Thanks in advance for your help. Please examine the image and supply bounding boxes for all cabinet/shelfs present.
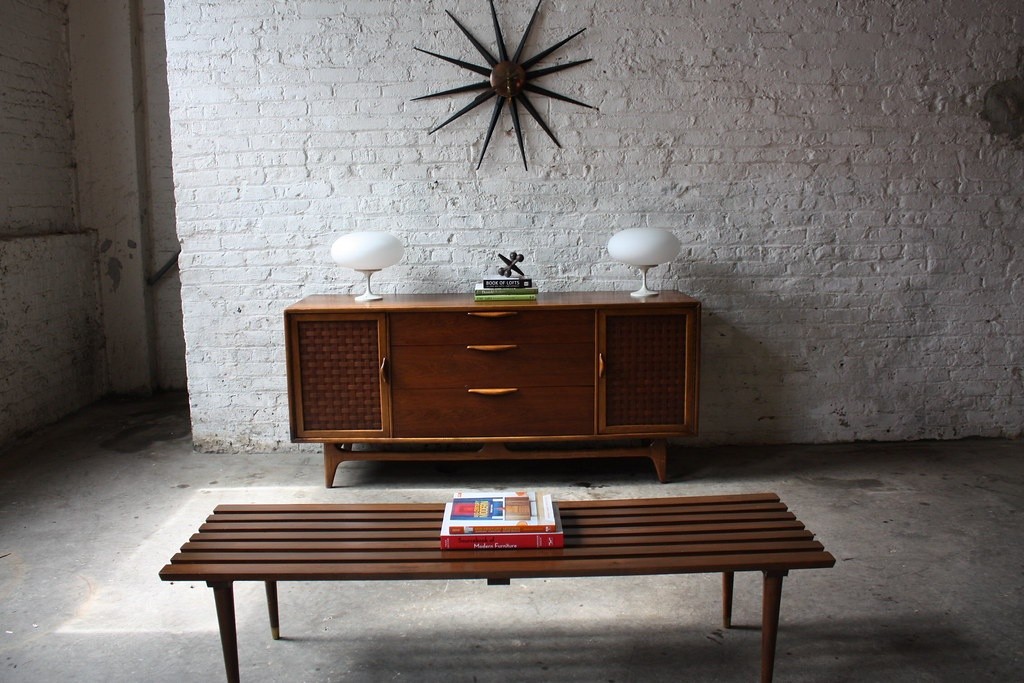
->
[283,291,703,488]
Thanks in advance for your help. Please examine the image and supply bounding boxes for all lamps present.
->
[608,228,681,297]
[331,231,404,302]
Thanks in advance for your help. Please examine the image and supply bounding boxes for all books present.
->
[474,275,539,302]
[440,491,565,550]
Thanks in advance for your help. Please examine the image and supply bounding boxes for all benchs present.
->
[159,492,835,683]
